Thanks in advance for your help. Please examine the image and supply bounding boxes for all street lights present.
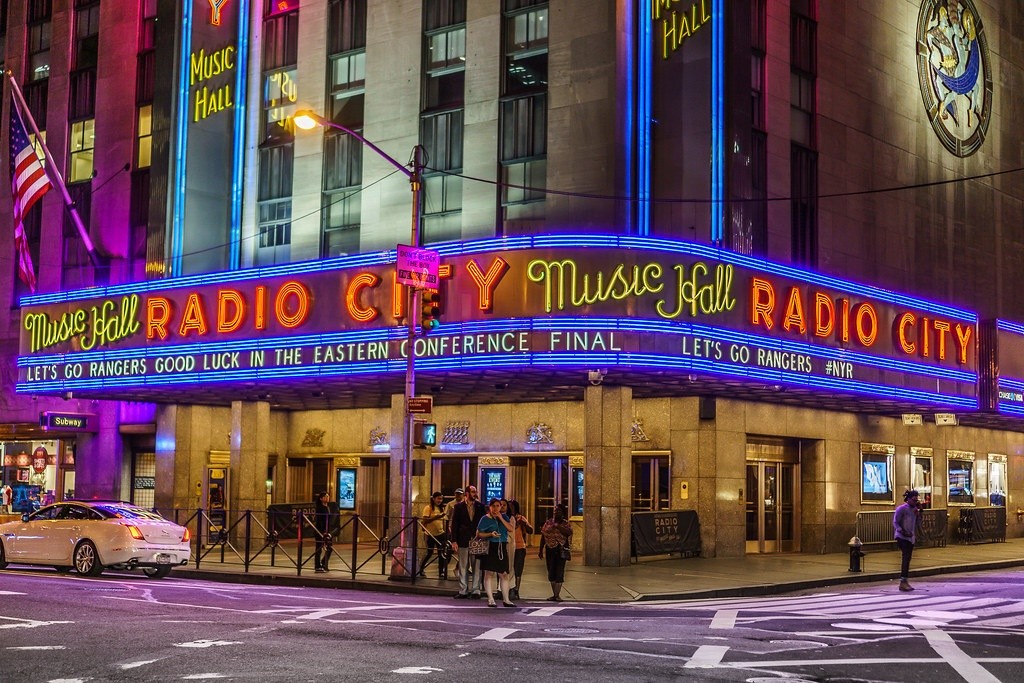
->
[292,107,425,583]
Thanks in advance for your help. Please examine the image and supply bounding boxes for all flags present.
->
[8,90,55,293]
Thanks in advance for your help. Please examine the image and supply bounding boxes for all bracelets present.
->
[919,509,924,512]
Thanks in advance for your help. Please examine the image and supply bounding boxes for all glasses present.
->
[909,497,919,503]
[470,490,479,493]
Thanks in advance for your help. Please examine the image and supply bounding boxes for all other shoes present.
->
[419,568,426,576]
[322,563,329,571]
[315,567,327,573]
[453,593,468,599]
[554,595,563,602]
[438,566,445,577]
[453,568,460,581]
[469,566,472,573]
[898,580,914,591]
[502,601,518,607]
[487,600,497,607]
[546,595,555,601]
[471,593,482,599]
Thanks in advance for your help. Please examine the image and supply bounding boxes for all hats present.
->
[454,488,464,494]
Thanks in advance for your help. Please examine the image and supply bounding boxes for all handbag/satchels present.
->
[561,548,572,562]
[468,532,490,556]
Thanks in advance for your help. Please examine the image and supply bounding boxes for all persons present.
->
[476,498,517,607]
[315,491,335,572]
[451,486,482,599]
[497,499,516,598]
[892,491,923,591]
[444,488,464,578]
[419,492,444,577]
[537,503,574,601]
[508,499,534,599]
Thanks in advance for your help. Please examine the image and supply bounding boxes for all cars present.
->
[0,501,192,579]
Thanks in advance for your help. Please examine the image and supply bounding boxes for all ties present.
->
[466,500,474,521]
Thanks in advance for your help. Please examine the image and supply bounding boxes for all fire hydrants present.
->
[847,535,867,573]
